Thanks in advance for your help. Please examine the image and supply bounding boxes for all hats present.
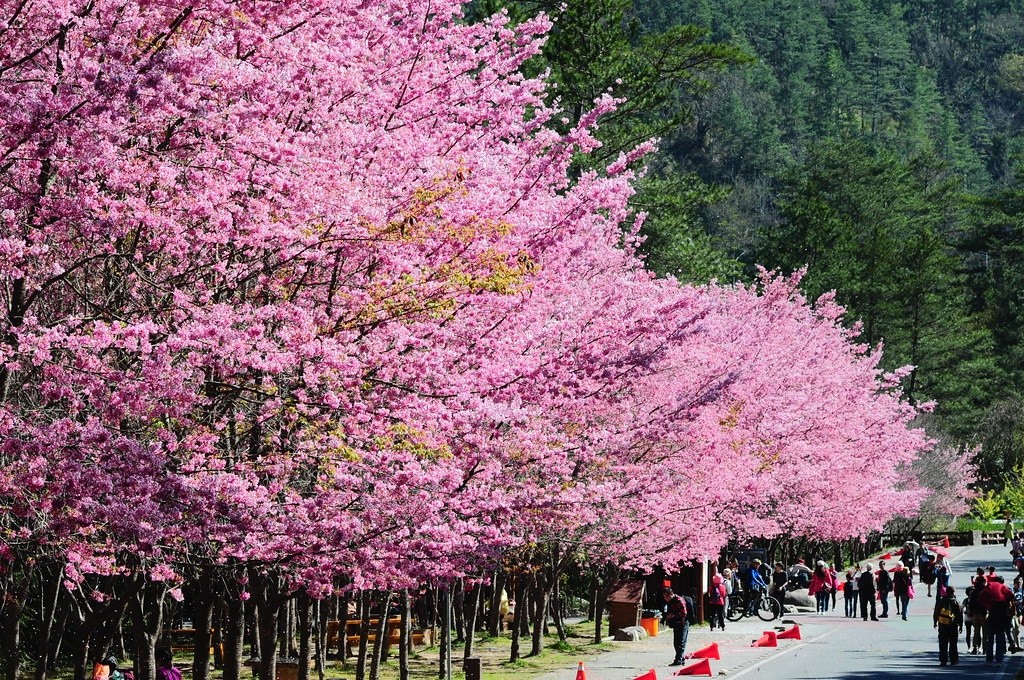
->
[866,563,873,570]
[753,558,759,562]
[986,565,995,572]
[902,567,911,575]
[799,559,805,563]
[722,568,731,575]
[817,560,824,566]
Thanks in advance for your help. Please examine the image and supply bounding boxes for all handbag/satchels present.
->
[908,586,914,599]
[824,583,830,593]
[91,662,110,680]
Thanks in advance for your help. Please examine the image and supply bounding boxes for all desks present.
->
[323,617,417,661]
[161,629,226,666]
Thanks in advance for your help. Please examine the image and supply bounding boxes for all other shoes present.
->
[864,617,867,621]
[878,614,888,618]
[871,616,879,621]
[902,616,907,621]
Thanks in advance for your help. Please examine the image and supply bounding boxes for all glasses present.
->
[753,562,760,565]
[878,564,883,566]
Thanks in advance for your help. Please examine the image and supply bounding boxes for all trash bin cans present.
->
[639,609,659,636]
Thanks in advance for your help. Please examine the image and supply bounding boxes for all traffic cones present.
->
[894,548,904,555]
[874,570,880,574]
[633,668,658,680]
[887,566,896,573]
[943,536,950,548]
[879,552,891,560]
[576,662,588,680]
[776,624,802,640]
[750,630,778,647]
[684,642,721,660]
[672,656,712,677]
[836,581,845,591]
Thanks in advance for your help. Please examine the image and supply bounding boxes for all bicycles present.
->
[725,586,781,622]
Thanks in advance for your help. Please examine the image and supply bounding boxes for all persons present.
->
[809,560,839,621]
[661,587,690,666]
[898,541,953,599]
[492,583,509,633]
[708,573,727,631]
[786,559,815,589]
[93,650,182,680]
[1004,518,1024,569]
[933,556,1024,666]
[718,558,788,629]
[843,560,915,621]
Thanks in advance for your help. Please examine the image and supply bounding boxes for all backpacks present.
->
[786,564,797,577]
[888,577,894,591]
[709,583,721,602]
[937,600,955,625]
[918,550,929,564]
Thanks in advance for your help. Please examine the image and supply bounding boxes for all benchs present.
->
[330,633,424,656]
[171,647,226,665]
